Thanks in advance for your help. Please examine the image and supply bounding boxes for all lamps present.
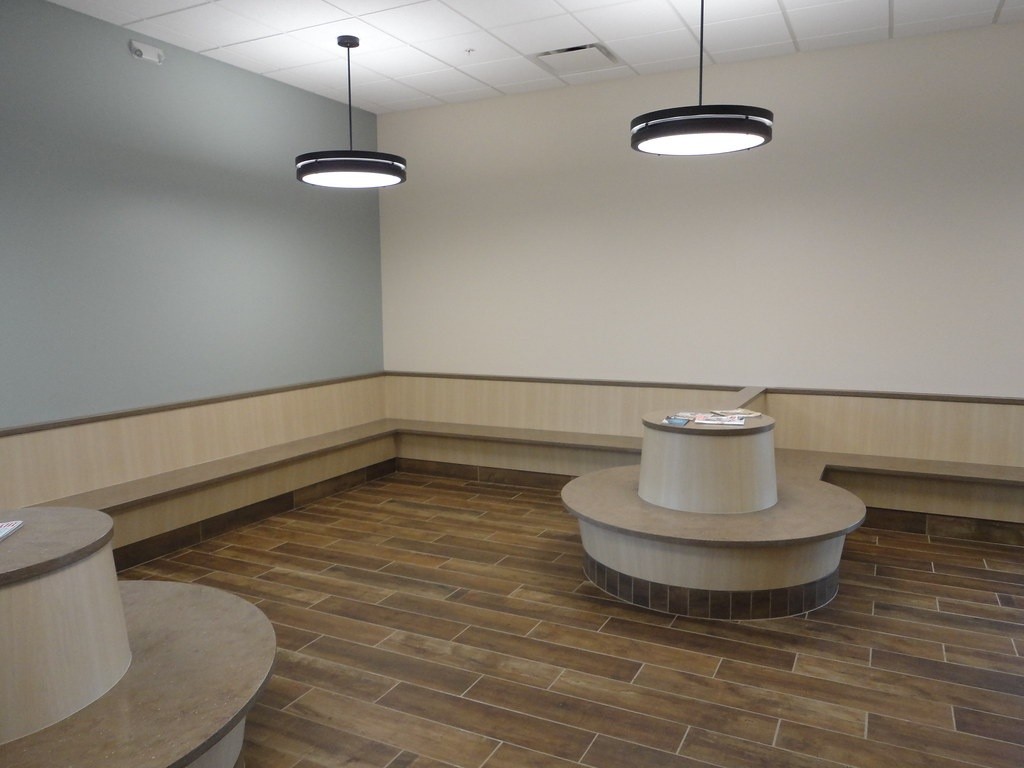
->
[294,35,406,188]
[629,0,773,154]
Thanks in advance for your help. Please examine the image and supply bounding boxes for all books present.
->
[0,521,24,544]
[656,407,763,426]
